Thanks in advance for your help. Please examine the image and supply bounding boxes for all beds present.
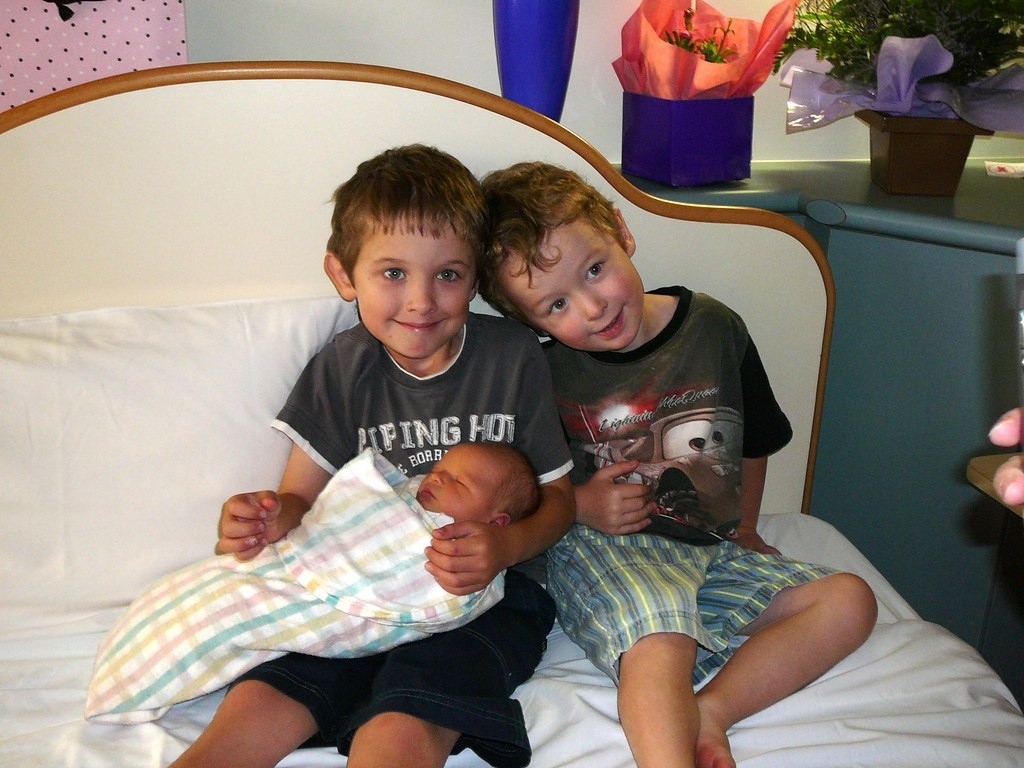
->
[0,62,1024,768]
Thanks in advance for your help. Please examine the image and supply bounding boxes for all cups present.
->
[1017,236,1024,407]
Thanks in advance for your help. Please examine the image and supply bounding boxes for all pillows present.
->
[0,296,362,606]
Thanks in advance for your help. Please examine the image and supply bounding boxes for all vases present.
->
[854,107,995,199]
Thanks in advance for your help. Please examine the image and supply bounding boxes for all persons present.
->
[480,162,879,767]
[989,407,1024,506]
[83,441,541,727]
[169,142,578,767]
